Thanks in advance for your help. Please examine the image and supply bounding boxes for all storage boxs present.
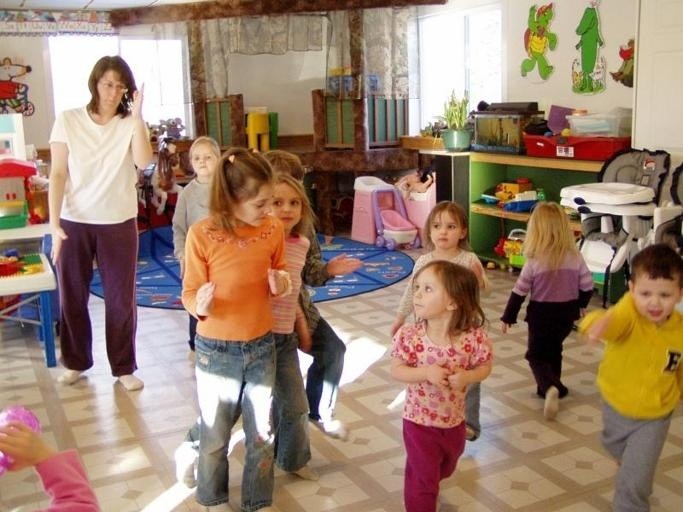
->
[521,129,632,162]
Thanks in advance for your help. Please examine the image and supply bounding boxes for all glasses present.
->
[94,78,130,94]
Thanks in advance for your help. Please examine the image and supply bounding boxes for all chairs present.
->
[639,164,682,272]
[559,147,670,306]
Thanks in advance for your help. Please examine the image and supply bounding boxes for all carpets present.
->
[90,226,415,311]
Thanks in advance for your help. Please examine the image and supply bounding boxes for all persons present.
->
[576,241,683,511]
[497,202,595,424]
[47,56,155,390]
[171,136,363,512]
[0,420,101,512]
[390,200,493,442]
[390,259,493,511]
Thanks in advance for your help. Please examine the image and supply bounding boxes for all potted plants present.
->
[443,89,472,151]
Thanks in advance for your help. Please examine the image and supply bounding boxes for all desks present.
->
[0,223,56,270]
[0,251,57,367]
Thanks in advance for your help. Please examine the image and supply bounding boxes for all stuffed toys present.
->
[146,118,185,216]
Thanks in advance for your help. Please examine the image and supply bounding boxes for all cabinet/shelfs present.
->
[468,153,630,305]
